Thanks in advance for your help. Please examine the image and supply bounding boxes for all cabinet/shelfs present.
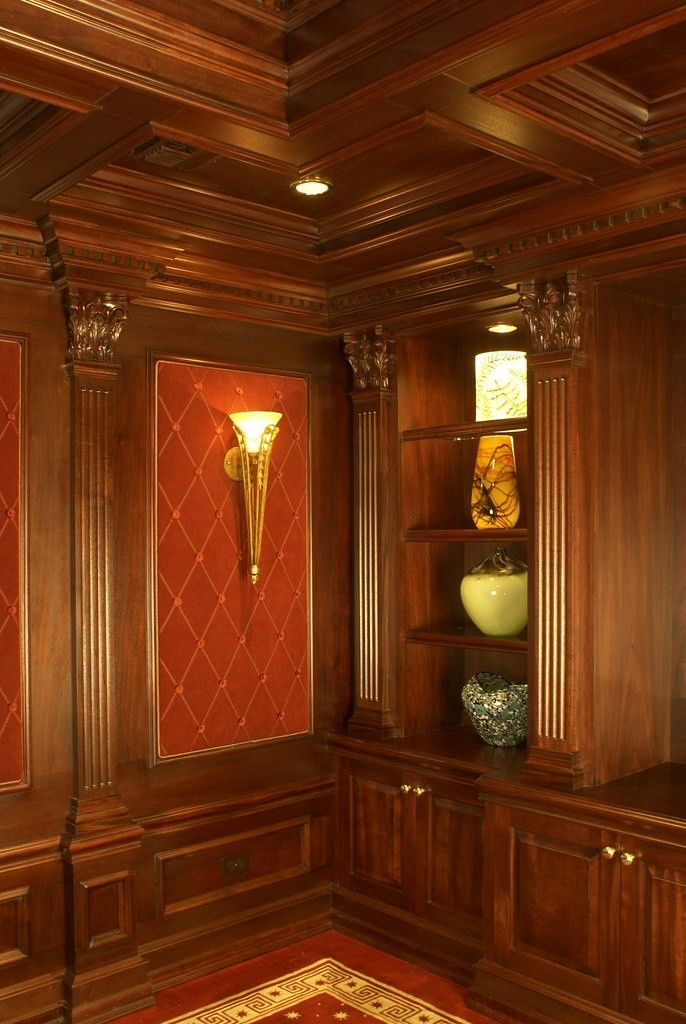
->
[401,418,530,653]
[324,729,526,986]
[466,762,686,1023]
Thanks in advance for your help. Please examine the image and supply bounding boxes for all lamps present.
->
[223,410,284,586]
